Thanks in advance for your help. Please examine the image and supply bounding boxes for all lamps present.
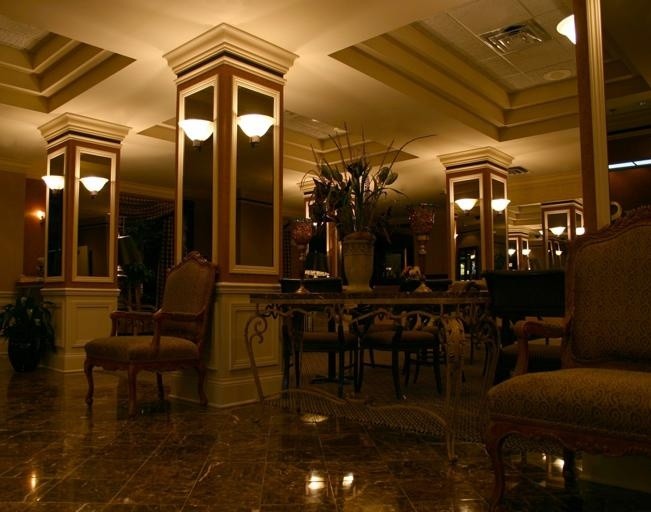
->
[455,198,478,215]
[508,226,585,256]
[80,176,110,199]
[178,118,214,149]
[556,14,576,45]
[40,175,65,196]
[236,113,274,142]
[608,156,651,173]
[491,198,511,215]
[37,210,46,226]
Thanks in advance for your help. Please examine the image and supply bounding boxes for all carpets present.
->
[263,365,651,458]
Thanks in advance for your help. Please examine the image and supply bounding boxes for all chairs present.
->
[279,268,569,399]
[84,252,220,421]
[482,205,651,512]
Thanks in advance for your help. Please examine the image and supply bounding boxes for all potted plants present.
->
[299,126,439,285]
[1,287,54,370]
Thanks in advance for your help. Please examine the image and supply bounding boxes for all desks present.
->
[244,292,487,422]
